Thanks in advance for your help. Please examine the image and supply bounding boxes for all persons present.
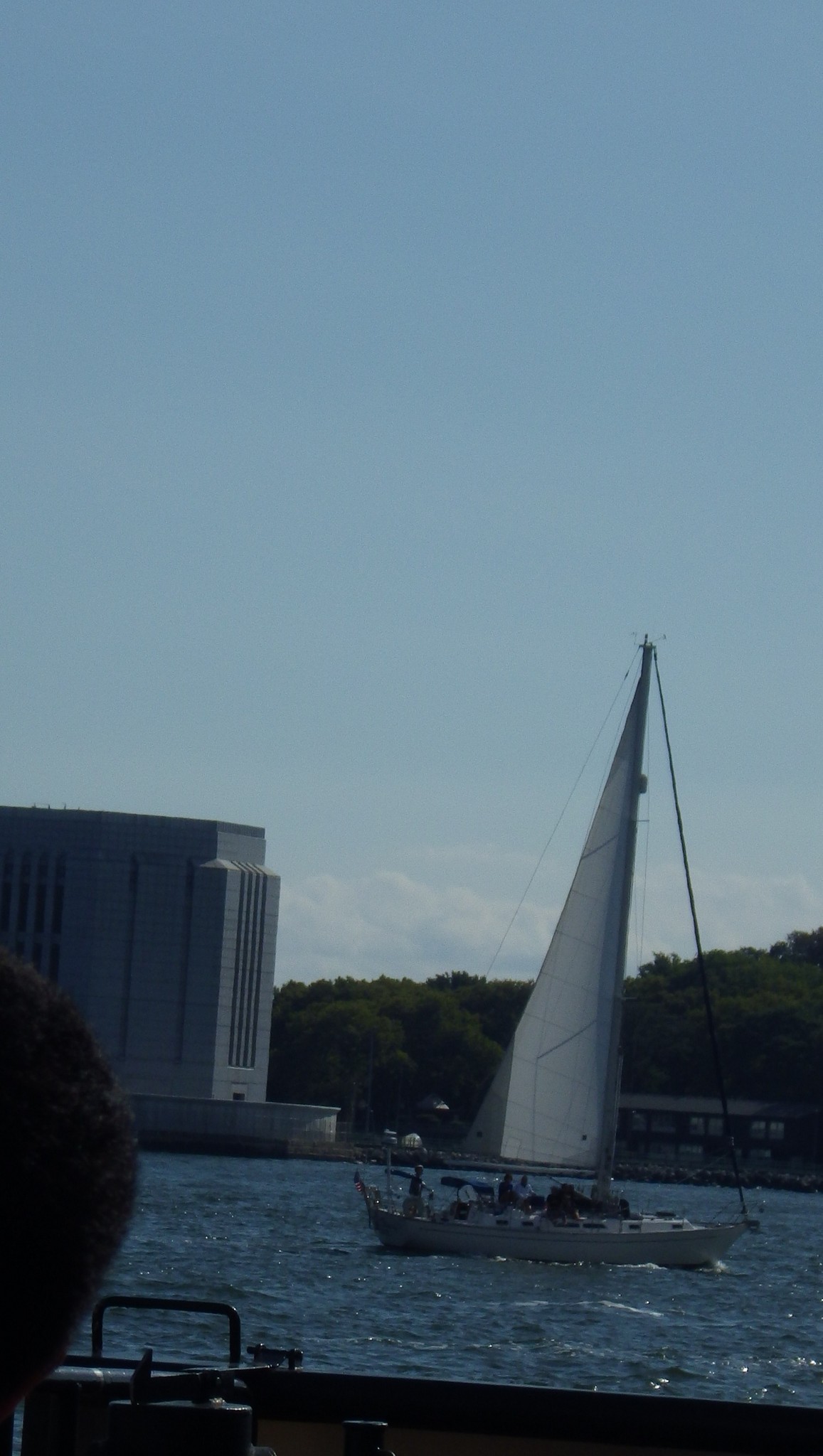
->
[396,1159,630,1229]
[0,943,142,1426]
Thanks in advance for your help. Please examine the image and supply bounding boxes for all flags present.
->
[353,1171,362,1192]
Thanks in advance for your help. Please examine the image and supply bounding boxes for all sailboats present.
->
[354,631,762,1270]
